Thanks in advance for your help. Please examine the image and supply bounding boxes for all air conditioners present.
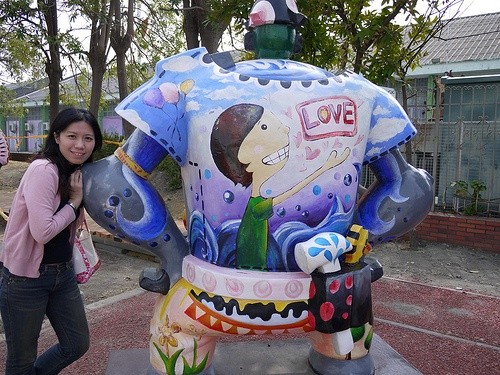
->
[9,123,17,131]
[25,122,33,131]
[42,122,50,130]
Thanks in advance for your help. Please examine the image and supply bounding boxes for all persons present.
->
[0,108,105,375]
